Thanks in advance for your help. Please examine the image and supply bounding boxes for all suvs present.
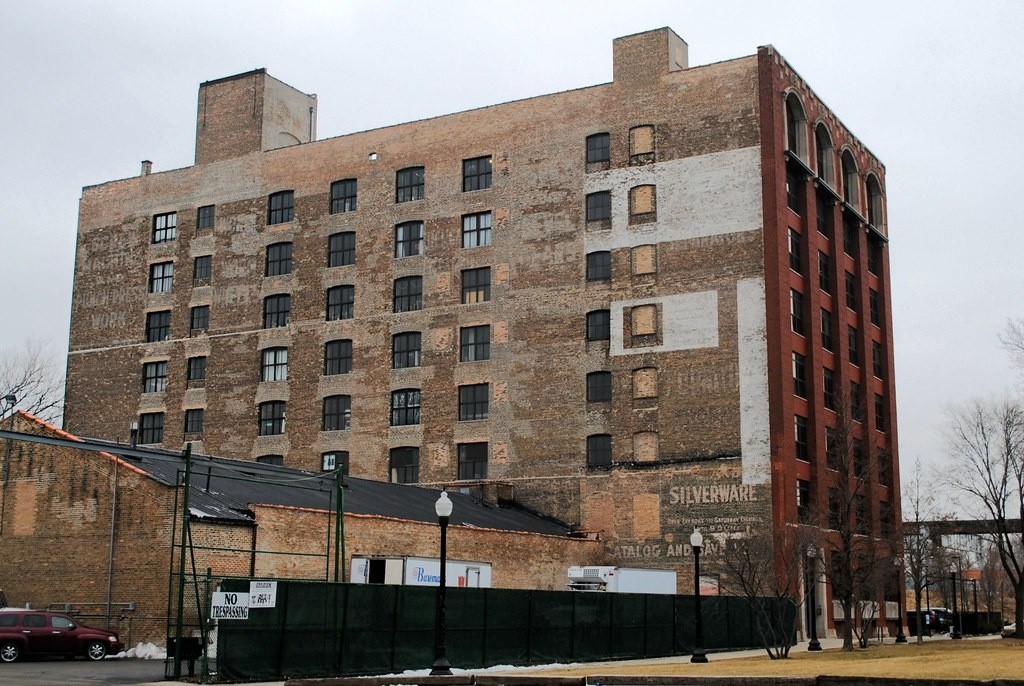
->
[0,607,126,664]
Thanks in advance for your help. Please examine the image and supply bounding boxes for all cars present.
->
[897,606,953,643]
[1001,620,1024,638]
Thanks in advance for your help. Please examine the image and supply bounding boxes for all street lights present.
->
[428,490,453,675]
[805,543,823,651]
[690,528,709,663]
[949,561,963,639]
